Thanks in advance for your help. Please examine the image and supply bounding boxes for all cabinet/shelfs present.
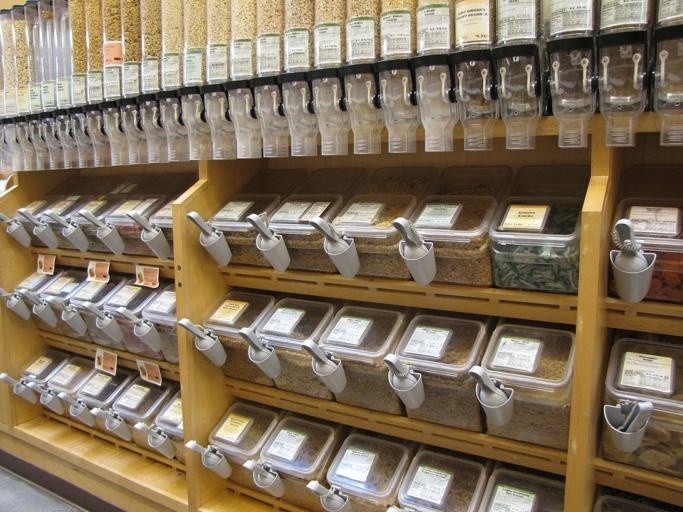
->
[1,106,683,512]
